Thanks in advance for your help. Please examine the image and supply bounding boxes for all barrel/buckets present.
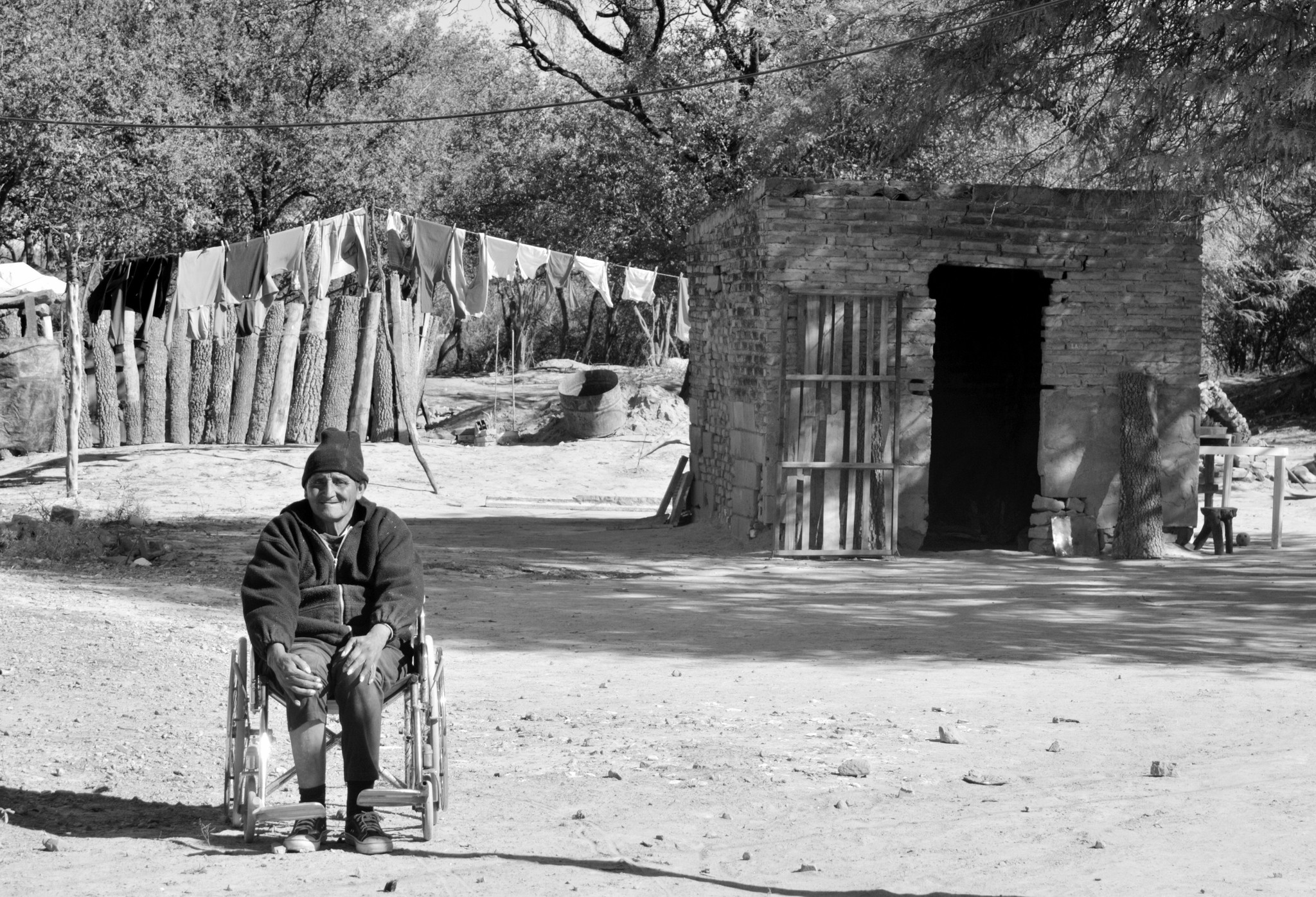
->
[558,369,626,438]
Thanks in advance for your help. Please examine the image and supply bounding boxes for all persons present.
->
[242,427,424,854]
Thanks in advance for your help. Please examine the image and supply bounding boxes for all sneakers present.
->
[283,815,328,852]
[345,811,393,855]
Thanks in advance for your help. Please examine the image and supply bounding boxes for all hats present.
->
[301,427,369,489]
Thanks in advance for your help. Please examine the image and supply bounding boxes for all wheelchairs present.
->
[224,595,448,840]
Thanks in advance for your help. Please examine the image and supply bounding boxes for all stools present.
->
[1193,507,1238,555]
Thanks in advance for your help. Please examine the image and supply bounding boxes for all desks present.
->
[1197,447,1289,548]
